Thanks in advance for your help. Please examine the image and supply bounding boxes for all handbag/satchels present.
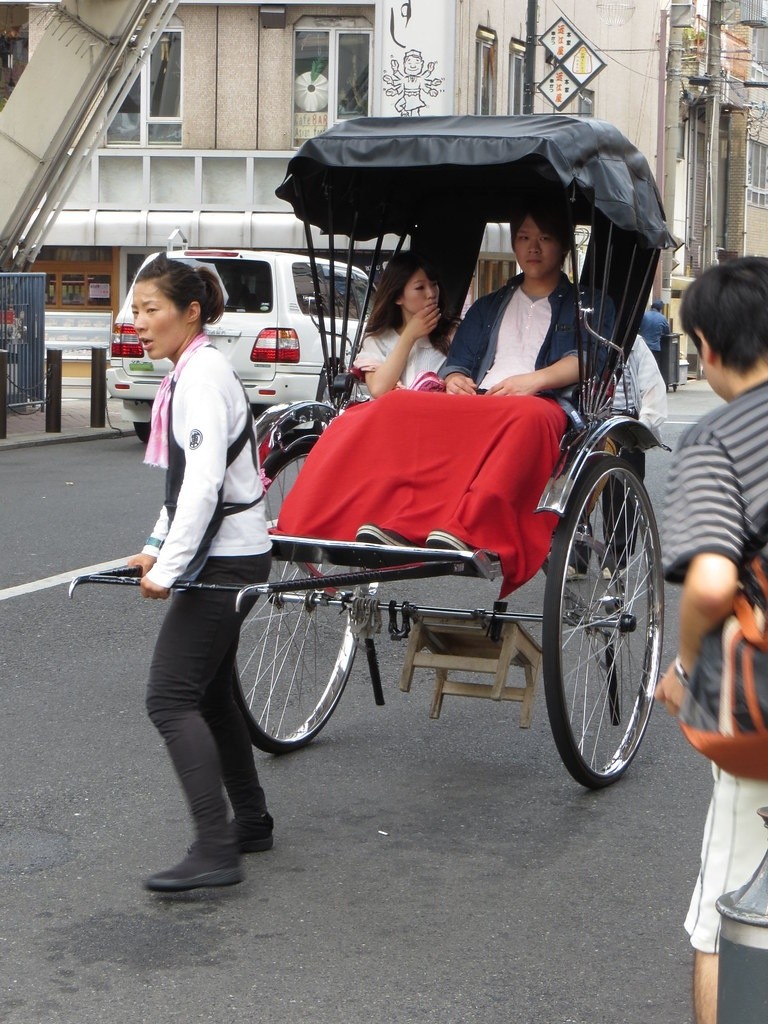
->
[677,519,767,780]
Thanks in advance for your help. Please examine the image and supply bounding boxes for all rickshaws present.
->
[69,111,672,795]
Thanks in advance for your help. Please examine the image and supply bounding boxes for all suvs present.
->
[103,249,381,445]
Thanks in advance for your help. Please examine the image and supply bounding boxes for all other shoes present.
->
[187,814,274,852]
[355,523,418,548]
[565,565,587,581]
[603,567,626,579]
[426,530,472,552]
[143,843,244,891]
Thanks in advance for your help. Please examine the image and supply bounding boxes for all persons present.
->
[354,251,457,399]
[355,199,582,556]
[543,332,667,581]
[650,256,768,1024]
[640,300,670,367]
[129,254,274,894]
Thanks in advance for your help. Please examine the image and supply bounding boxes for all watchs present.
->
[675,657,688,686]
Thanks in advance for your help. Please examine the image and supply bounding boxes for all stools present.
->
[398,615,544,730]
